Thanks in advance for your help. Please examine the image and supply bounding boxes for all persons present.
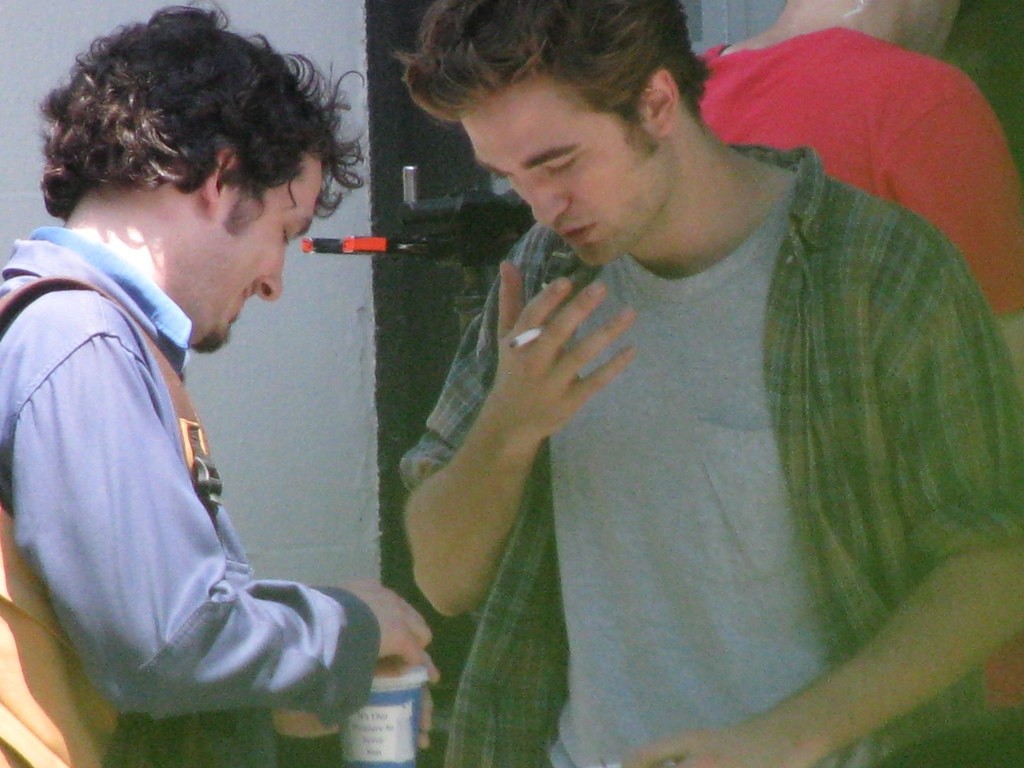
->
[698,0,1024,768]
[401,1,1022,768]
[0,0,439,768]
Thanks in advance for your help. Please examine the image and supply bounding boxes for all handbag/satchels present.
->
[0,278,222,768]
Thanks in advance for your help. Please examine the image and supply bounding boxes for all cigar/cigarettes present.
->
[511,323,549,349]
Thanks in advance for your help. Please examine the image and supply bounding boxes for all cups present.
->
[339,666,426,768]
